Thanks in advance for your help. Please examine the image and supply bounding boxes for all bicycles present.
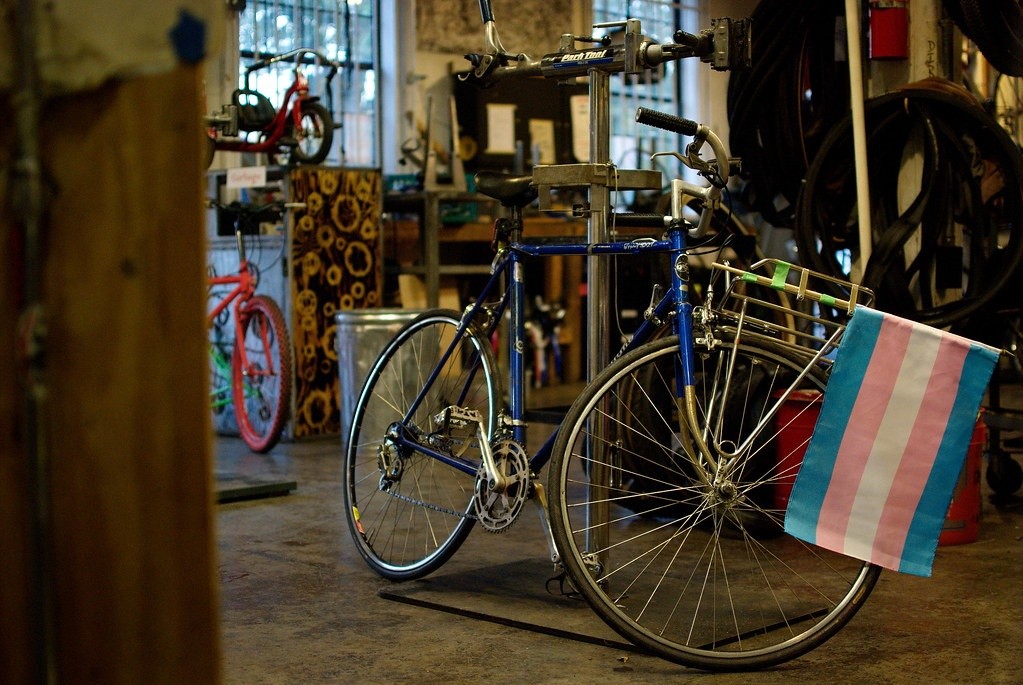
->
[203,195,303,452]
[346,108,888,668]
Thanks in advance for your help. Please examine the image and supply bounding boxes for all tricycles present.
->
[204,48,337,170]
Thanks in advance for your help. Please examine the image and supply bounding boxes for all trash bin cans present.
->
[936,407,988,546]
[772,388,825,516]
[332,307,443,460]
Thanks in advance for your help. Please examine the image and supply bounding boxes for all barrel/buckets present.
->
[336,303,449,458]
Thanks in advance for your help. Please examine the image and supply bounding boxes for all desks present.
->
[379,212,667,385]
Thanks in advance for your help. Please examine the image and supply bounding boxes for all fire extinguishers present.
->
[868,0,910,59]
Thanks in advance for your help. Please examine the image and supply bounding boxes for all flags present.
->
[783,306,999,579]
